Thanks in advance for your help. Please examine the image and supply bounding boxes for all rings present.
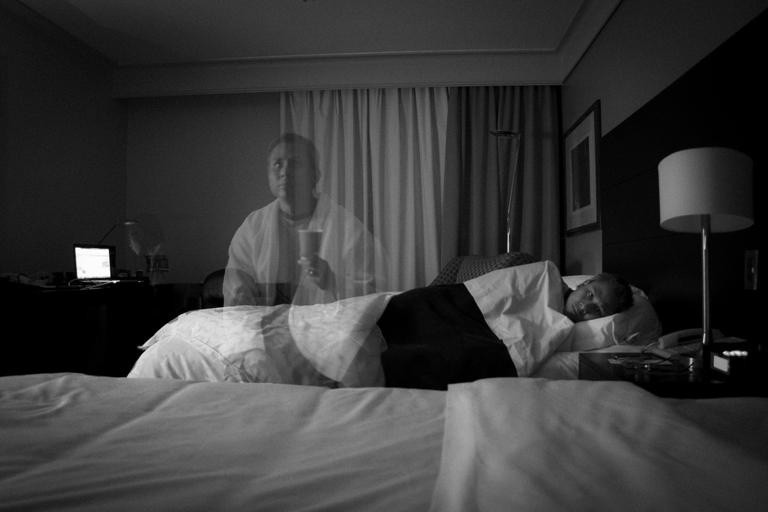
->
[309,268,317,276]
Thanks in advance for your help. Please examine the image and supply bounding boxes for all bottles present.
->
[144,224,170,285]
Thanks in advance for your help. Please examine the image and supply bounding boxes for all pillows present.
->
[561,275,661,352]
[562,99,602,237]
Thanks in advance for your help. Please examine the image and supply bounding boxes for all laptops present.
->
[73,244,145,285]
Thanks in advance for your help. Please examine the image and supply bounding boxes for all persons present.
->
[221,131,390,386]
[560,272,634,322]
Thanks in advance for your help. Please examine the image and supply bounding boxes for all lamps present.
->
[657,148,753,369]
[98,220,138,245]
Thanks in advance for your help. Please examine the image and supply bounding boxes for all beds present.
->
[0,372,768,512]
[126,260,663,389]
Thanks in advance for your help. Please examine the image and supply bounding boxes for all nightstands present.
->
[578,352,768,398]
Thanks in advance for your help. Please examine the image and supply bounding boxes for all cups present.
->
[295,228,323,260]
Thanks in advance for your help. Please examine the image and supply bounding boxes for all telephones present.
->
[647,328,746,359]
